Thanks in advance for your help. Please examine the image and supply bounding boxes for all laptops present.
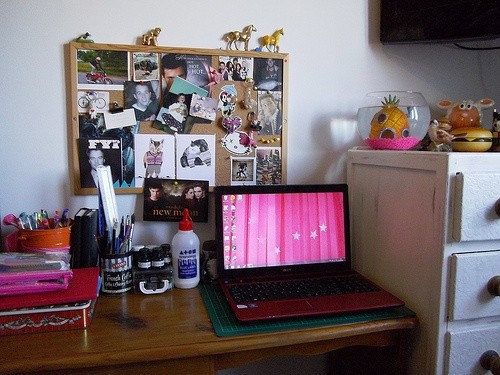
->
[214,184,407,322]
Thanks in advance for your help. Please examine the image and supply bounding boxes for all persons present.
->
[145,181,207,217]
[84,146,117,189]
[259,93,281,136]
[87,55,105,76]
[260,59,280,90]
[161,92,189,134]
[130,82,156,120]
[220,57,248,82]
[159,52,187,89]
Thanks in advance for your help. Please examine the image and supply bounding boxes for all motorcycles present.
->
[86,70,114,85]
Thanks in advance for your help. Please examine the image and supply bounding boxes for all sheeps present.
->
[435,98,495,128]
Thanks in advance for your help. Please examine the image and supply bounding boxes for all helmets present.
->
[96,57,101,60]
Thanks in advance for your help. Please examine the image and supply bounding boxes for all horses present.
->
[226,24,257,51]
[259,28,285,53]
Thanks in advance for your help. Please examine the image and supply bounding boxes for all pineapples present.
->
[367,95,409,140]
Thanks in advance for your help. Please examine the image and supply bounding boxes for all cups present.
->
[18,218,73,254]
[100,247,133,297]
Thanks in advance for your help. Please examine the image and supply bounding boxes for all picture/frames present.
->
[67,41,286,197]
[143,177,209,223]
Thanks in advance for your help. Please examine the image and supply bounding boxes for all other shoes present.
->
[91,73,96,75]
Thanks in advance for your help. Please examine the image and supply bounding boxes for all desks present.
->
[0,287,421,375]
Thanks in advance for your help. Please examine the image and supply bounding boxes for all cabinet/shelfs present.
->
[346,150,500,375]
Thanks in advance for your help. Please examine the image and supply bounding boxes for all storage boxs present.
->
[0,292,99,337]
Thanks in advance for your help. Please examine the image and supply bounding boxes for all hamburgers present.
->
[449,128,493,151]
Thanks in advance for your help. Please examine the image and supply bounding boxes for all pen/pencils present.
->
[2,207,73,229]
[92,213,136,254]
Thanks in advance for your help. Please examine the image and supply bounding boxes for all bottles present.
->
[201,240,218,284]
[132,244,171,270]
[171,208,200,288]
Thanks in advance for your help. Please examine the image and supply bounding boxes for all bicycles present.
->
[78,92,106,109]
